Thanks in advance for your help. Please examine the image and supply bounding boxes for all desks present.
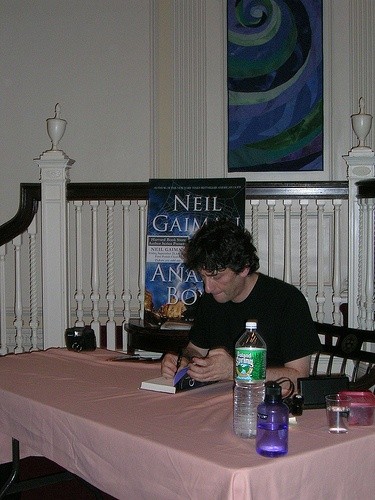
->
[0,339,375,500]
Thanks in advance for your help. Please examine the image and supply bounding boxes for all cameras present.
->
[65,327,96,353]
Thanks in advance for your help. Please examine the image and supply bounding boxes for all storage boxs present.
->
[338,391,375,427]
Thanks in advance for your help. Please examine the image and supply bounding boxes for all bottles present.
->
[233,322,267,437]
[256,379,294,457]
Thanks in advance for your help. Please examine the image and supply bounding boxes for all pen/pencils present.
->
[174,353,182,376]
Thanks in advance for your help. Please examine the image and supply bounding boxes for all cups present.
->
[325,394,352,434]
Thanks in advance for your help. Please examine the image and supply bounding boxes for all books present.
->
[141,357,219,395]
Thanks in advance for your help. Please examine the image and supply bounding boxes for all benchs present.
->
[309,321,375,396]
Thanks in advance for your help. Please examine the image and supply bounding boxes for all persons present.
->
[161,218,323,399]
